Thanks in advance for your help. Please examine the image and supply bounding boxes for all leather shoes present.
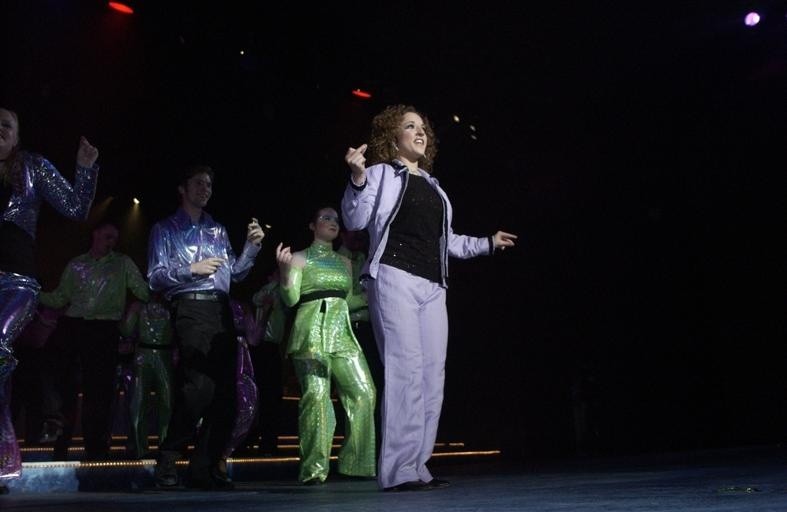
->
[419,477,451,490]
[382,481,433,493]
[153,465,178,487]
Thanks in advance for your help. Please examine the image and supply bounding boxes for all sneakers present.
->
[188,460,235,493]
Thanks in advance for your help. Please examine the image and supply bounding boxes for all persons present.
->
[273,206,380,484]
[337,104,519,493]
[15,215,376,478]
[146,157,269,490]
[0,102,102,496]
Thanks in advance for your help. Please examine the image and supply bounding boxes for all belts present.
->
[173,290,229,303]
